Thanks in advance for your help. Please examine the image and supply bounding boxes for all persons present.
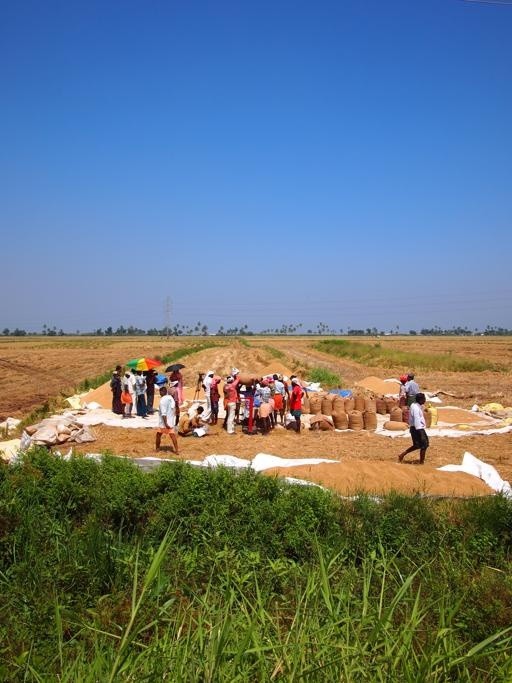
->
[109,365,309,456]
[398,376,409,408]
[397,392,430,464]
[403,372,421,408]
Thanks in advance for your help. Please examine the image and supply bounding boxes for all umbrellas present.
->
[124,357,164,375]
